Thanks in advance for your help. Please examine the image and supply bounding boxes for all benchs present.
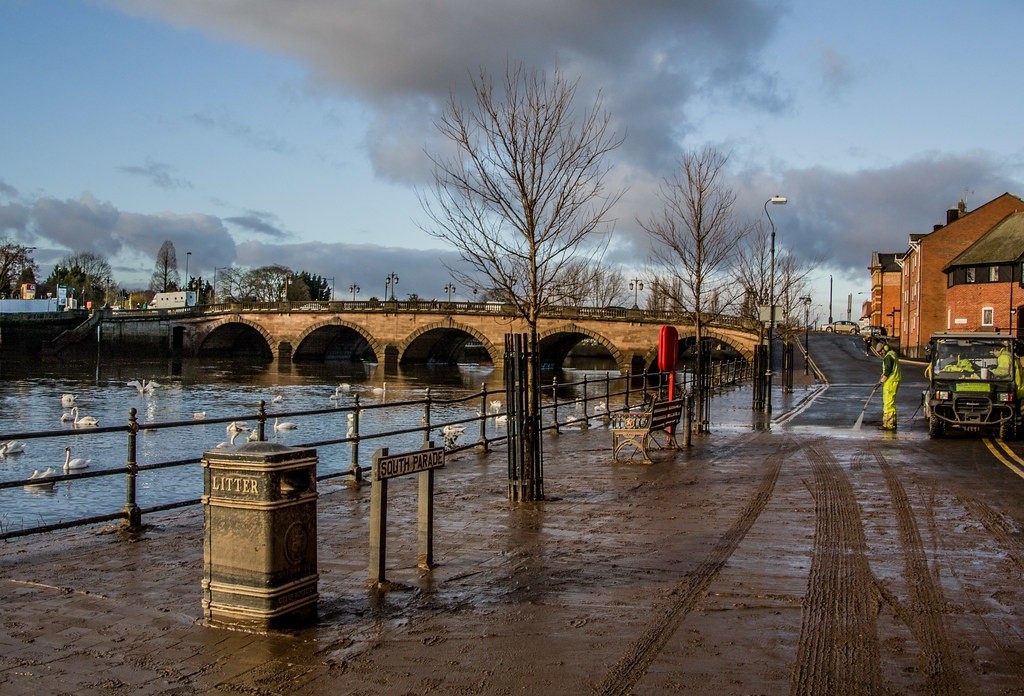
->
[613,389,689,464]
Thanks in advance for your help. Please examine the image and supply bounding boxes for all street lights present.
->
[629,277,644,310]
[803,294,812,375]
[349,283,360,301]
[443,282,456,303]
[20,246,36,283]
[186,252,192,290]
[213,267,233,303]
[322,276,334,300]
[763,195,788,389]
[386,272,400,301]
[280,272,294,301]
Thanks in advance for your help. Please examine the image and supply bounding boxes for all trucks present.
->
[922,331,1019,442]
[146,291,196,311]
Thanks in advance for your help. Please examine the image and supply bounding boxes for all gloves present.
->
[874,381,883,390]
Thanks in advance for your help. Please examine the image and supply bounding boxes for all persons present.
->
[934,352,975,376]
[136,302,148,314]
[876,341,901,431]
[988,344,1024,443]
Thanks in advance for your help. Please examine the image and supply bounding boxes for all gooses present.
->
[193,411,207,420]
[216,421,268,449]
[70,406,99,425]
[329,382,351,399]
[345,402,364,444]
[565,396,643,426]
[61,406,79,422]
[443,399,507,448]
[0,440,26,454]
[62,393,79,408]
[63,447,91,469]
[25,467,57,487]
[127,379,161,397]
[273,418,298,430]
[273,395,283,403]
[373,381,387,391]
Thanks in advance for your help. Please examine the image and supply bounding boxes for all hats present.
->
[874,342,885,352]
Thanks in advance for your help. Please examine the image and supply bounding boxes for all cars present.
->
[821,321,860,336]
[859,325,887,337]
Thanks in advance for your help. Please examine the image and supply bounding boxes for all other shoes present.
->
[878,425,895,430]
